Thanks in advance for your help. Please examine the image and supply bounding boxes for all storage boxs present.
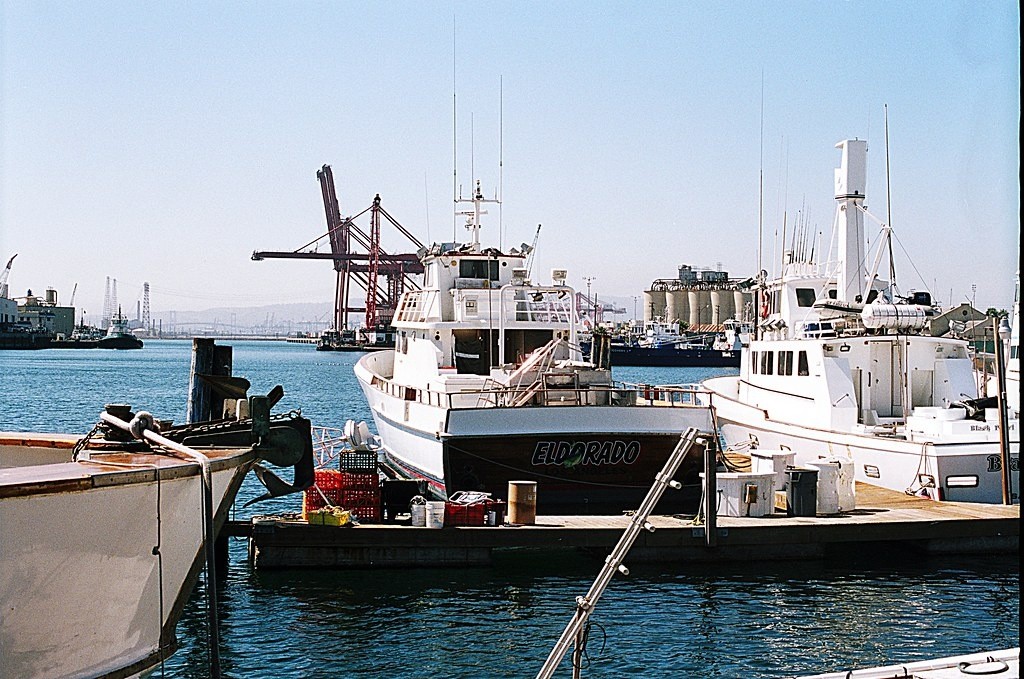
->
[302,450,382,524]
[384,480,428,521]
[444,501,505,527]
[699,472,776,515]
[751,445,795,491]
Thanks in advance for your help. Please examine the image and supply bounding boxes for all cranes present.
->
[251,164,429,330]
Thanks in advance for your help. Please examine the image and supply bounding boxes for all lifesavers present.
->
[760,291,769,317]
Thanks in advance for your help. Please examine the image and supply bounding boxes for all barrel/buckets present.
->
[425,500,445,528]
[507,480,537,526]
[487,501,506,526]
[784,455,856,518]
[412,505,426,526]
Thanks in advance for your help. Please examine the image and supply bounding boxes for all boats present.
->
[0,386,315,679]
[354,12,1019,517]
[51,304,144,349]
